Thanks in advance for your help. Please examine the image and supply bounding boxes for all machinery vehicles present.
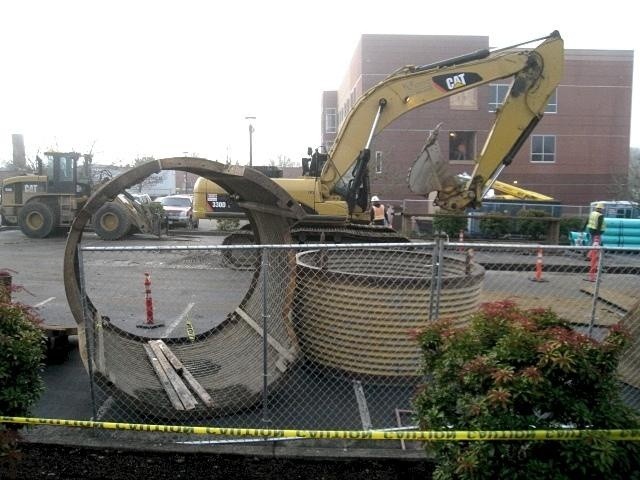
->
[452,172,557,202]
[0,147,169,240]
[194,29,566,272]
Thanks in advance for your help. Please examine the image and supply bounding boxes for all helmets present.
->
[371,196,381,202]
[595,202,604,209]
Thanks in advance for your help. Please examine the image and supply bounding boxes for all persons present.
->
[579,203,609,262]
[457,139,467,160]
[367,194,390,228]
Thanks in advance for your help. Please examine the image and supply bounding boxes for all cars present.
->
[159,192,199,231]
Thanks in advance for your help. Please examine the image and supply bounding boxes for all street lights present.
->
[243,116,257,168]
